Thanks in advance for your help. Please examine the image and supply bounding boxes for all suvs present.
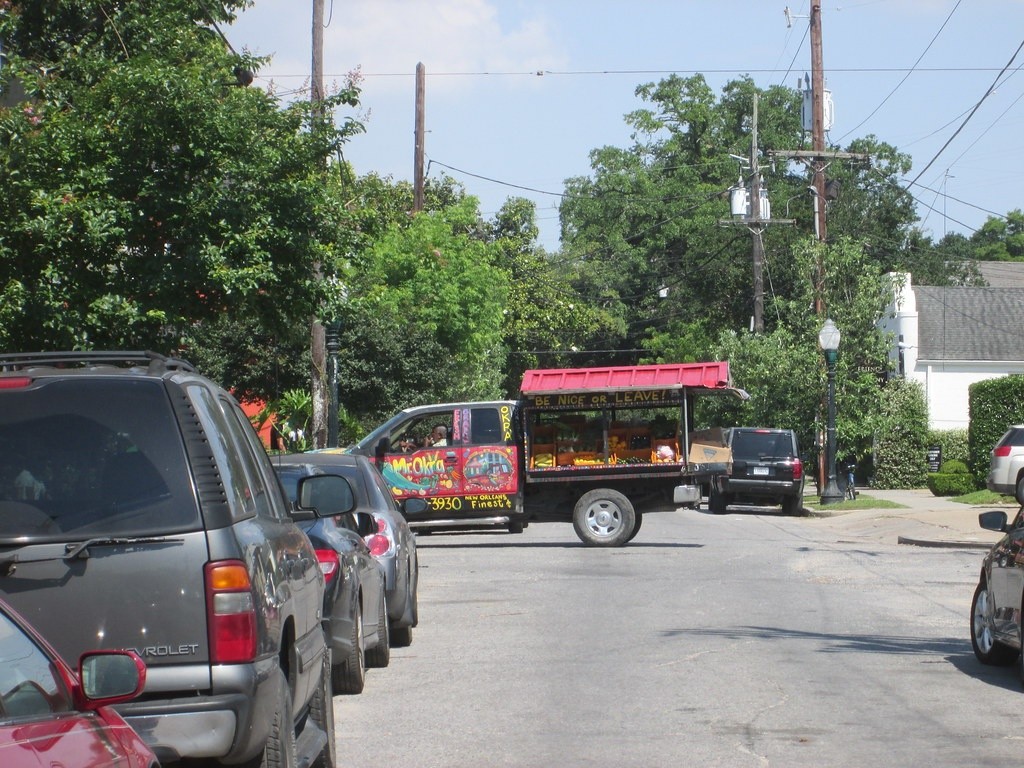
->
[707,426,805,517]
[1,348,336,768]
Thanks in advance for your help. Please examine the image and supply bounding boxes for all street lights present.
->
[817,316,845,505]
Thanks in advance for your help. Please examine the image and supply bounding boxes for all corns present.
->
[535,452,552,467]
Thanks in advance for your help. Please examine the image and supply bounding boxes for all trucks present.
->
[335,360,751,549]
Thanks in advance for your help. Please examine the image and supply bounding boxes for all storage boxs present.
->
[530,414,680,470]
[689,425,733,474]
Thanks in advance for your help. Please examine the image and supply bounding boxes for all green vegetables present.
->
[558,428,577,441]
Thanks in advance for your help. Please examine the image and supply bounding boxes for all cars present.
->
[970,505,1024,666]
[0,597,161,768]
[988,424,1024,507]
[265,452,430,648]
[270,462,390,696]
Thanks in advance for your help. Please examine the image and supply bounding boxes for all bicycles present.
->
[841,460,859,500]
